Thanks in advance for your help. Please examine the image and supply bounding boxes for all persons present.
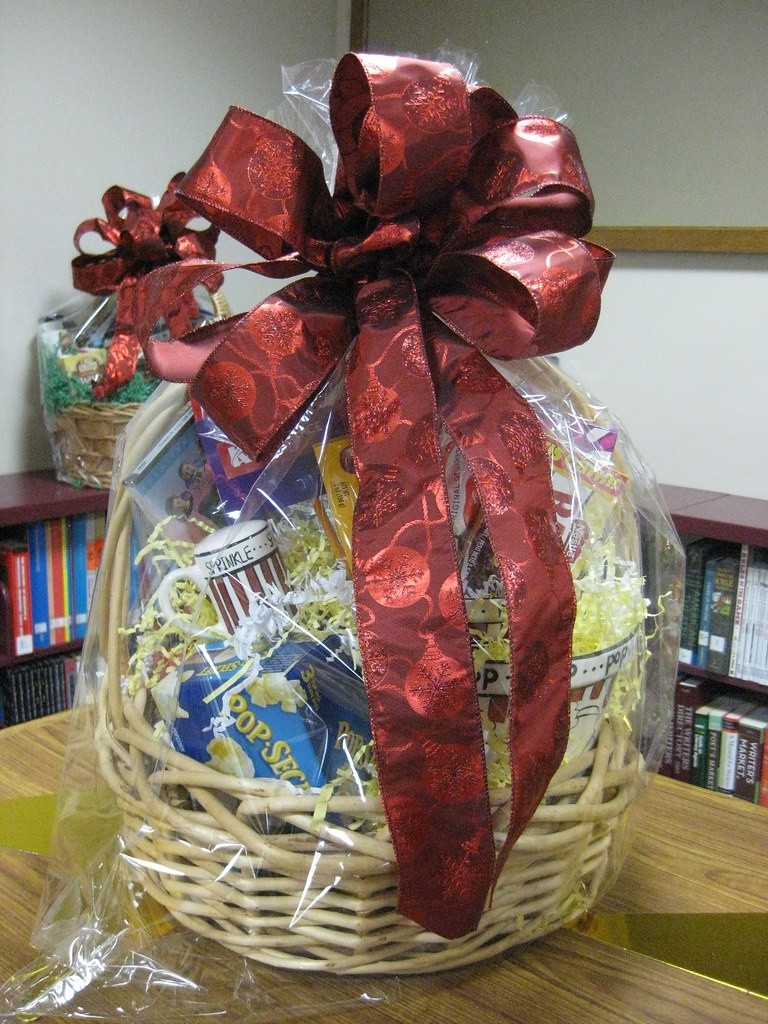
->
[178,463,215,494]
[166,496,193,521]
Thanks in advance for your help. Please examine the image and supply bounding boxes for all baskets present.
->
[109,339,650,974]
[40,288,229,490]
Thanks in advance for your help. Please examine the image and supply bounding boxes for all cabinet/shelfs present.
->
[0,467,767,804]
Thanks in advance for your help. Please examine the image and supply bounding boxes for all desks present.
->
[1,705,768,1024]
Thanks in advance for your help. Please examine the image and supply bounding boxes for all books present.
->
[640,539,768,807]
[0,510,109,725]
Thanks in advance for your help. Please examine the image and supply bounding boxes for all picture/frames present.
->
[348,0,768,254]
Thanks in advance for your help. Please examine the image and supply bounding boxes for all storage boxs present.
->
[39,315,172,397]
[153,639,374,834]
